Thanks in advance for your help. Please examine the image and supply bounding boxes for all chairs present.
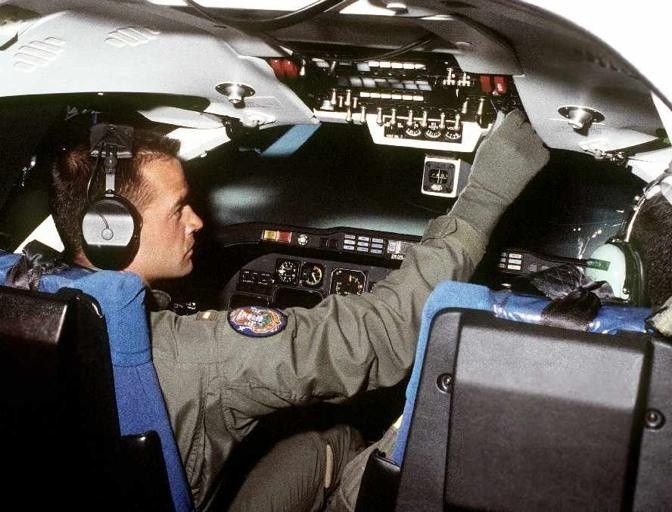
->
[0,252,197,511]
[351,281,671,512]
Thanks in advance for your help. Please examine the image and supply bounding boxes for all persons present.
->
[49,109,550,511]
[326,174,672,512]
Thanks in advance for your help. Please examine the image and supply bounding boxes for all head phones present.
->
[78,122,144,271]
[584,162,672,308]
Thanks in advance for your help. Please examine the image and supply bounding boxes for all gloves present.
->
[447,110,551,233]
